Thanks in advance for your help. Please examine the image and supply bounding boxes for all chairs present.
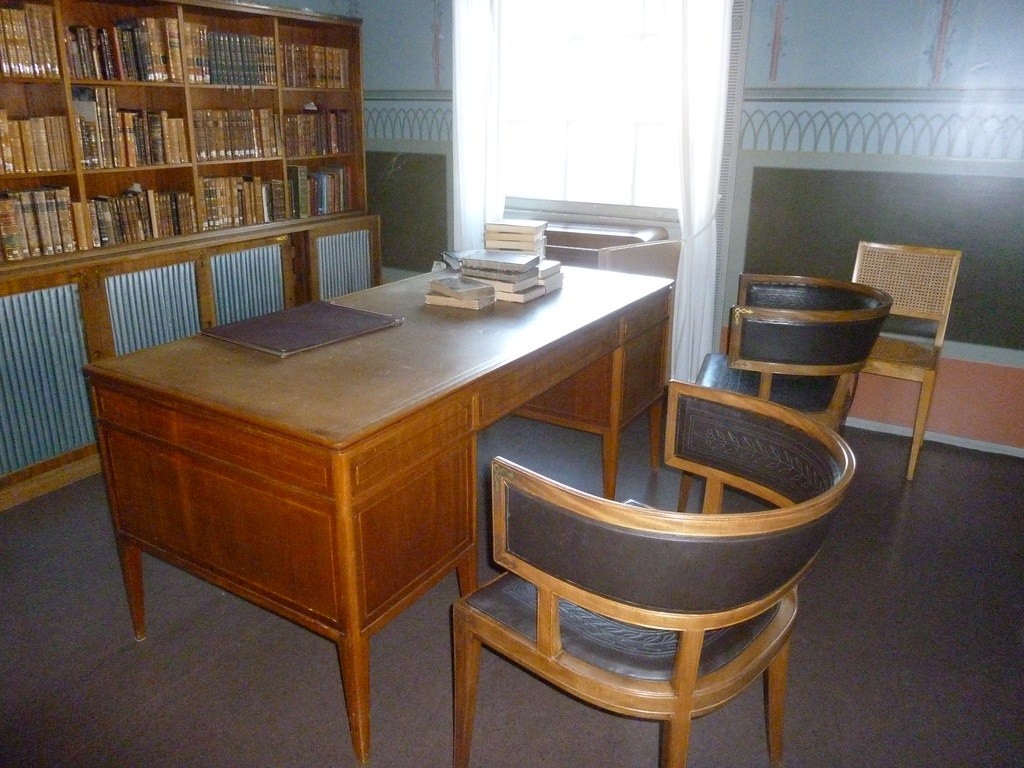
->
[677,272,894,515]
[447,378,857,767]
[845,240,962,479]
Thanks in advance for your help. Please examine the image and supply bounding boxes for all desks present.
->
[79,249,672,768]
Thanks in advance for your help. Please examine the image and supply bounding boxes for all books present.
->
[1,159,358,272]
[0,84,358,181]
[1,2,352,90]
[423,217,565,311]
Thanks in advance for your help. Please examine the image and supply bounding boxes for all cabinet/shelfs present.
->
[0,0,383,514]
